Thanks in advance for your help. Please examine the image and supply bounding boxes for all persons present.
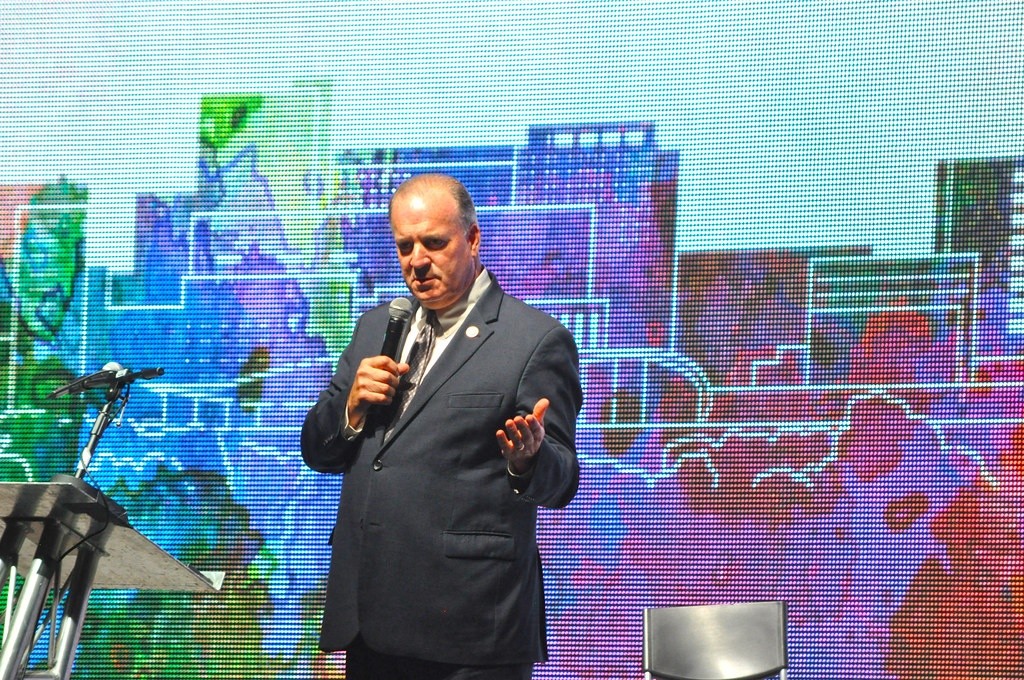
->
[300,173,583,680]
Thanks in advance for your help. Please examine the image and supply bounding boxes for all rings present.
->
[518,445,524,451]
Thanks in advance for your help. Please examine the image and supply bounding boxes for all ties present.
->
[384,311,439,448]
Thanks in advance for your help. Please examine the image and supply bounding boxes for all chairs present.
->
[641,601,788,680]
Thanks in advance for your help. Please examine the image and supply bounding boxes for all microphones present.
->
[51,362,124,397]
[380,298,414,360]
